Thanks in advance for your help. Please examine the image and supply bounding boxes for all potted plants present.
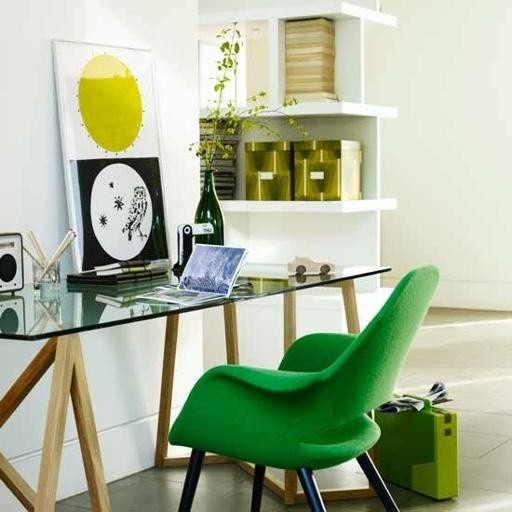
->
[189,16,317,251]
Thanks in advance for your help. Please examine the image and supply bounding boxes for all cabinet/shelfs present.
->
[191,1,406,402]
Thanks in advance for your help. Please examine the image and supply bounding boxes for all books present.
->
[285,18,335,98]
[67,243,246,307]
[199,120,241,200]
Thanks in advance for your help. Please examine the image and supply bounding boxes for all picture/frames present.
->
[73,287,182,331]
[49,35,176,277]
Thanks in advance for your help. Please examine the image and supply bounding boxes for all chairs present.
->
[155,262,445,512]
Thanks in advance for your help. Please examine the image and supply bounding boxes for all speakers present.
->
[0,231,25,294]
[0,297,27,337]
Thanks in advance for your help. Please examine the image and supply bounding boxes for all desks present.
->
[0,254,393,511]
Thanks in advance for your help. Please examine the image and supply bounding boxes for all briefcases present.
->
[370,393,459,502]
